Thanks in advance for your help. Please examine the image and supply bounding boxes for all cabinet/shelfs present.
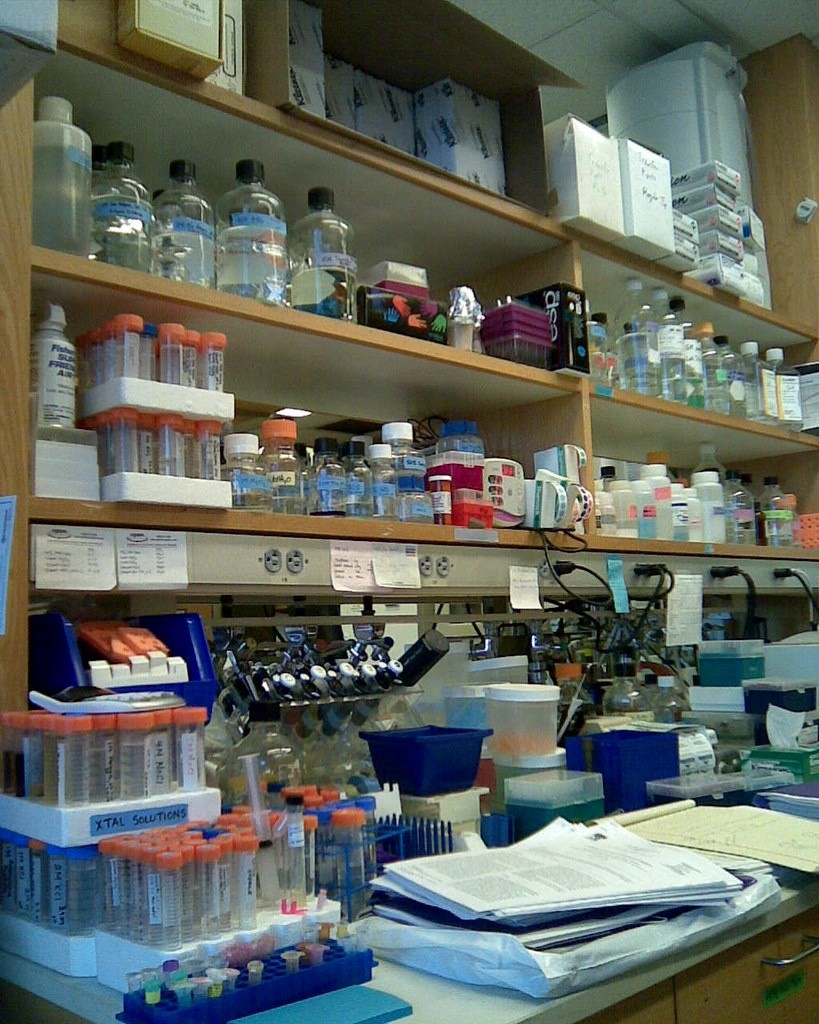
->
[0,31,819,750]
[0,877,819,1024]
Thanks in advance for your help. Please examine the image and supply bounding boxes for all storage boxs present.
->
[688,686,746,713]
[698,639,765,688]
[516,281,591,379]
[743,676,816,713]
[542,113,767,304]
[504,770,605,841]
[682,710,766,748]
[359,725,494,797]
[243,0,585,215]
[535,444,585,534]
[646,767,795,808]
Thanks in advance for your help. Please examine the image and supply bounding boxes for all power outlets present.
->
[539,560,550,576]
[265,548,304,573]
[420,556,450,576]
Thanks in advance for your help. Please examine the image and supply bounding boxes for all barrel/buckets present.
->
[404,642,566,815]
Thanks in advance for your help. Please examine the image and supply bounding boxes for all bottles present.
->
[652,675,691,724]
[591,279,804,433]
[221,418,485,524]
[215,158,288,309]
[30,96,93,260]
[92,141,216,290]
[288,186,357,319]
[595,440,801,545]
[602,645,654,725]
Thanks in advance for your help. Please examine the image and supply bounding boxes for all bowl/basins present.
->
[359,725,494,797]
